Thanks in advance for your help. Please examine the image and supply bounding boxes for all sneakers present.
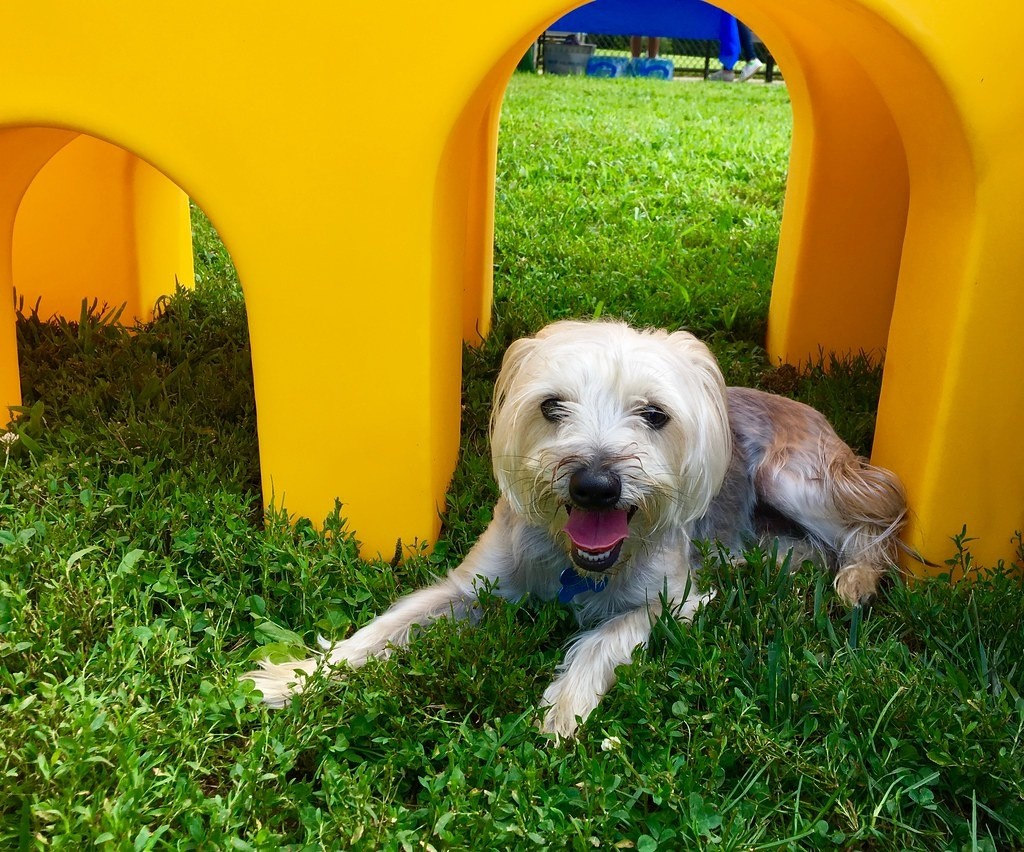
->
[735,58,761,82]
[707,70,735,81]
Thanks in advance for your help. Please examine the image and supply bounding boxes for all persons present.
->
[630,35,659,60]
[703,6,762,83]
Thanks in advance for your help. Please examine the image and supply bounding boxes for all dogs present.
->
[221,318,910,738]
